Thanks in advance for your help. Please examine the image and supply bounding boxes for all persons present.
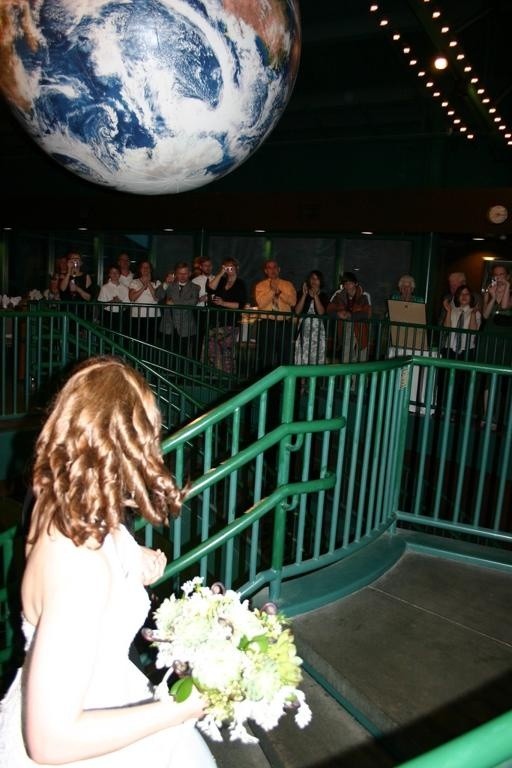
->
[327,273,373,397]
[0,355,220,768]
[441,285,482,423]
[254,259,298,374]
[386,273,425,302]
[294,270,329,395]
[437,271,468,326]
[40,250,248,381]
[482,264,512,430]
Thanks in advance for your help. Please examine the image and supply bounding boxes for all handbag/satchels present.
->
[338,271,358,285]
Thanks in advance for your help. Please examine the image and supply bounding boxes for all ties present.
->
[271,293,281,313]
[455,311,465,353]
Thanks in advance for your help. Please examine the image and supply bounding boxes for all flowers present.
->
[141,574,314,746]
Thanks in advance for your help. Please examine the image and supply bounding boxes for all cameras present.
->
[224,266,232,272]
[492,276,497,287]
[72,259,81,269]
